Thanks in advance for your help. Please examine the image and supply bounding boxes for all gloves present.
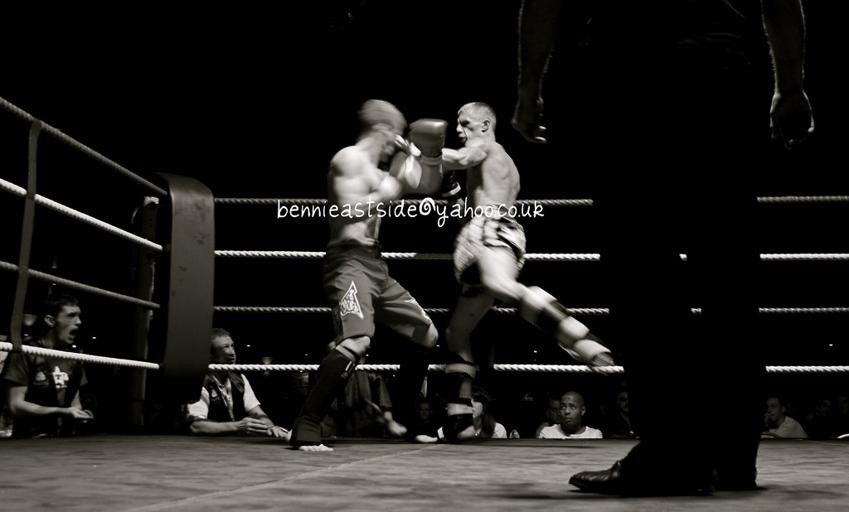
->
[408,118,448,166]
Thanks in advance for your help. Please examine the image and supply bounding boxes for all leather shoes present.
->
[569,458,756,496]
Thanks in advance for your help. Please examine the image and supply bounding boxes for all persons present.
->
[288,98,446,456]
[509,1,819,500]
[0,294,848,443]
[380,99,619,445]
